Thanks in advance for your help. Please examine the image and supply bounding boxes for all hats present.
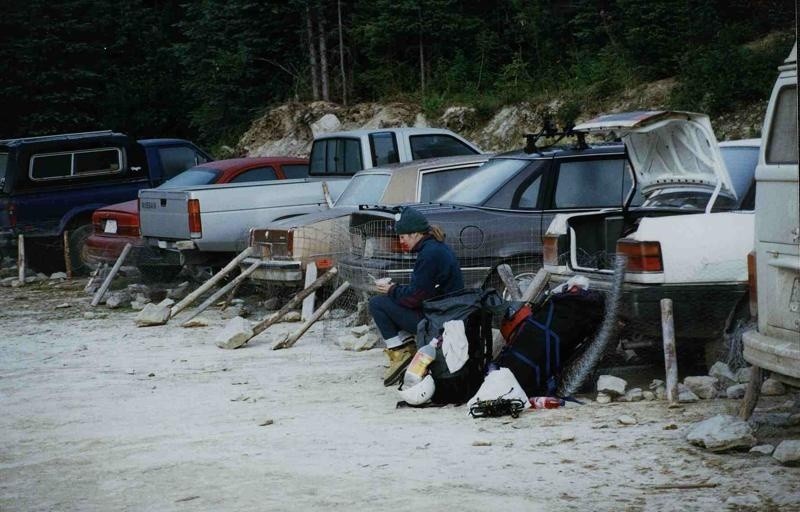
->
[395,206,431,235]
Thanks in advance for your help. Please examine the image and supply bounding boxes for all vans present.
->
[738,38,800,385]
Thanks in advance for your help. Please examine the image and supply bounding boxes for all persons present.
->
[367,205,468,388]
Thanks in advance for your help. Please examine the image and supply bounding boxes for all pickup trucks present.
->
[137,127,485,308]
[0,130,215,278]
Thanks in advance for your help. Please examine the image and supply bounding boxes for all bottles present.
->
[530,395,565,409]
[403,336,439,386]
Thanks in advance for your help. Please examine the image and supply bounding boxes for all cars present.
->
[238,152,492,308]
[80,157,310,283]
[329,143,631,300]
[542,111,762,373]
[540,108,762,376]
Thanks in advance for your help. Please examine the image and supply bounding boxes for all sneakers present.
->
[407,342,417,356]
[383,349,413,387]
